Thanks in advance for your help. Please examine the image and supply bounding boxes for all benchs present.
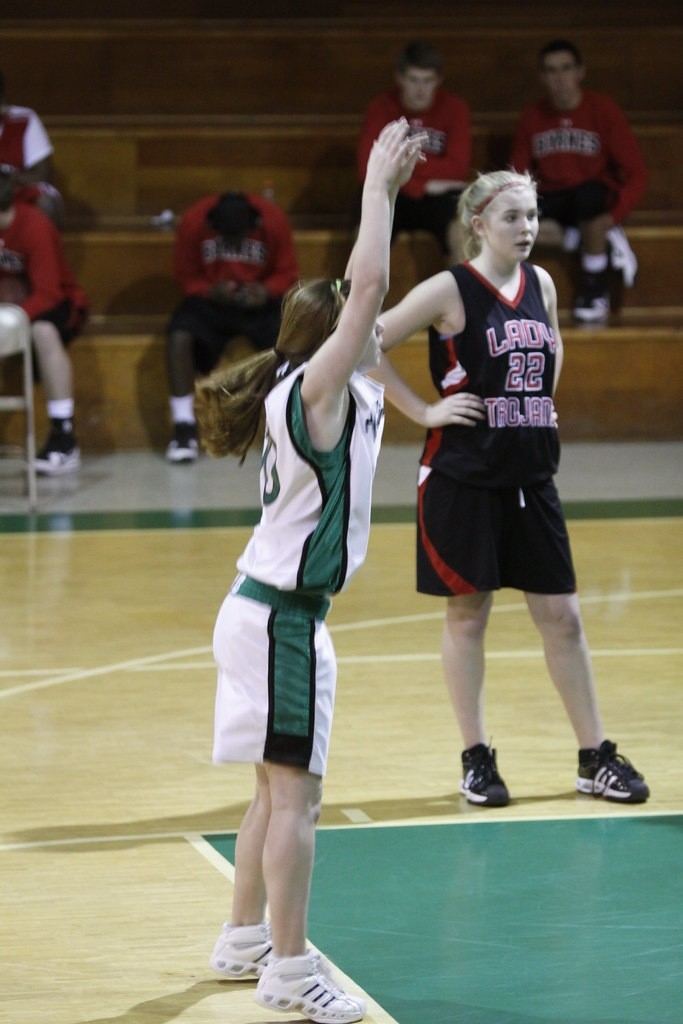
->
[0,0,682,453]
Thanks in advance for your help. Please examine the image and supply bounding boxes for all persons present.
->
[0,73,62,229]
[162,190,301,464]
[372,172,650,807]
[512,38,648,324]
[210,118,429,1023]
[352,41,471,270]
[0,170,90,476]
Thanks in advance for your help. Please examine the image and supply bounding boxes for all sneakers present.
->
[575,739,650,803]
[458,742,509,807]
[212,921,321,980]
[252,950,368,1024]
[165,421,199,461]
[36,436,80,475]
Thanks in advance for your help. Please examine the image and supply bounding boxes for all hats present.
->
[204,187,263,233]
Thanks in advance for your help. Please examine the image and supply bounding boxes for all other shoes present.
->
[573,280,610,319]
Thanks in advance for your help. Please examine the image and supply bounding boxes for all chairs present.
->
[0,300,38,515]
